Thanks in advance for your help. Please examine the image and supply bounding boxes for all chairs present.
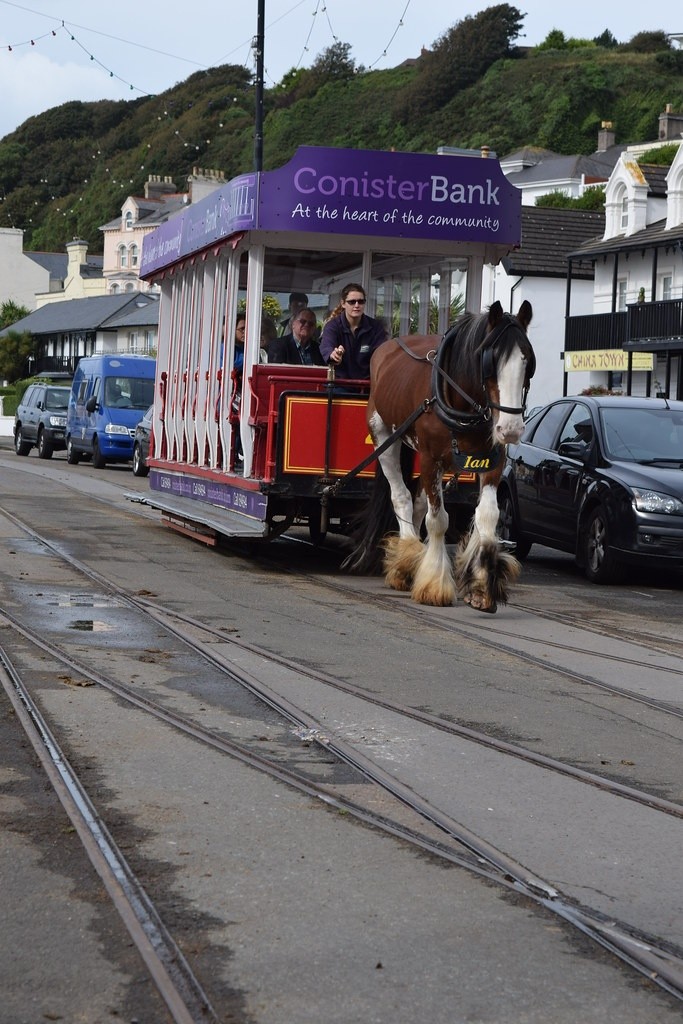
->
[110,384,122,401]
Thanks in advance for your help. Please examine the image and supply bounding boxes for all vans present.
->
[65,353,157,469]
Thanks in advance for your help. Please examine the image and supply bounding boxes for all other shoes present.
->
[234,454,243,468]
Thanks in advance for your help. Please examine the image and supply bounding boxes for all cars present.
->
[132,403,157,477]
[503,394,683,583]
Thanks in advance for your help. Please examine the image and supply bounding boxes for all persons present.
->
[222,292,328,366]
[109,384,122,403]
[318,283,388,381]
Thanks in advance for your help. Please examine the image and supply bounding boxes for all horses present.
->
[367,300,536,614]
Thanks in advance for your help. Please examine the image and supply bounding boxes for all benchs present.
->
[248,363,330,480]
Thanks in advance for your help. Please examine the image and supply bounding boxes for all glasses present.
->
[346,299,366,305]
[296,319,316,327]
[236,328,245,332]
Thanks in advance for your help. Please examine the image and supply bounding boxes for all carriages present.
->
[123,151,536,617]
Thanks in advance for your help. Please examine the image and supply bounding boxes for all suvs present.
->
[12,382,72,459]
